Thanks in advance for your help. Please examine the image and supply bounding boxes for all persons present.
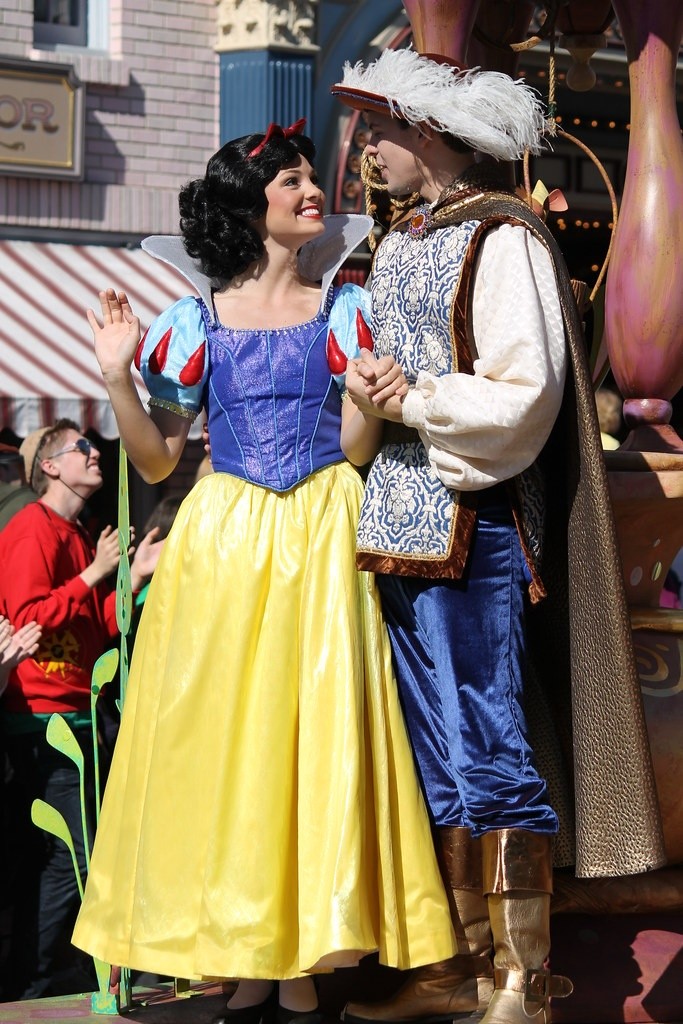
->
[0,417,214,1005]
[70,128,459,1024]
[328,53,666,1023]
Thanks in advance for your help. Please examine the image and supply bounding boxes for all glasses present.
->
[46,438,96,456]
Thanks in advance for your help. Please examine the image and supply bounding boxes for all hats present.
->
[330,48,545,159]
[19,426,55,483]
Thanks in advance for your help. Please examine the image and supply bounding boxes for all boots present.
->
[477,829,573,1024]
[345,826,495,1024]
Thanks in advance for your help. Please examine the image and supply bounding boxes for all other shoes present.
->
[211,980,278,1024]
[277,974,323,1024]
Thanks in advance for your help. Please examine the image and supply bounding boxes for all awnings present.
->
[0,239,212,439]
